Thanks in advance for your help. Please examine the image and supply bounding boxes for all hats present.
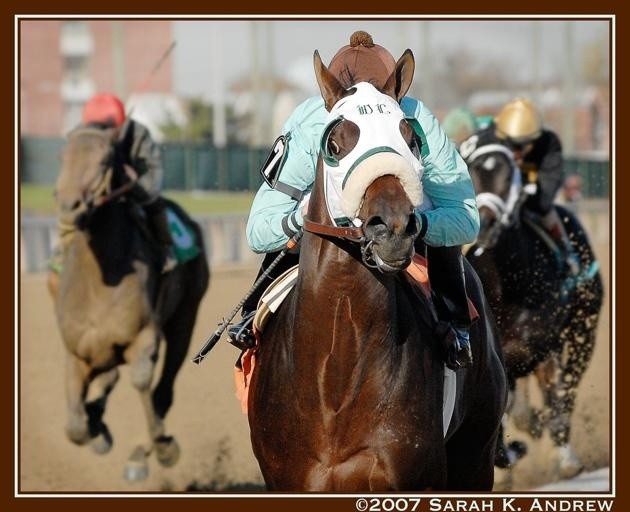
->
[496,98,542,143]
[84,94,125,126]
[326,31,396,92]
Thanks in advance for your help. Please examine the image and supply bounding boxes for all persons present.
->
[76,93,169,363]
[235,29,481,367]
[455,95,581,279]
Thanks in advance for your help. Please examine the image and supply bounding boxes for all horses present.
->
[455,120,605,478]
[245,47,509,493]
[55,118,211,484]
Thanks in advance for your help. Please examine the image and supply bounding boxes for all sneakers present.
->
[228,313,253,343]
[457,332,473,365]
